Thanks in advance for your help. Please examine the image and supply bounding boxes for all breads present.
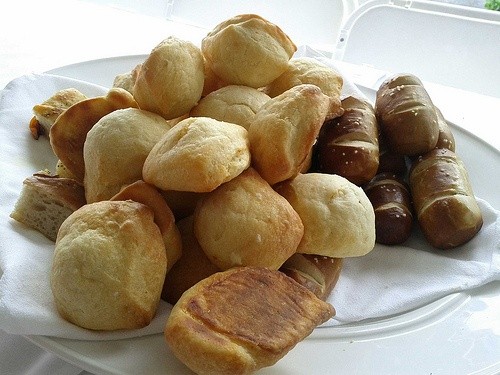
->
[6,13,480,375]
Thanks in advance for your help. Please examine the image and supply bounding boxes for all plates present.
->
[0,54,500,375]
